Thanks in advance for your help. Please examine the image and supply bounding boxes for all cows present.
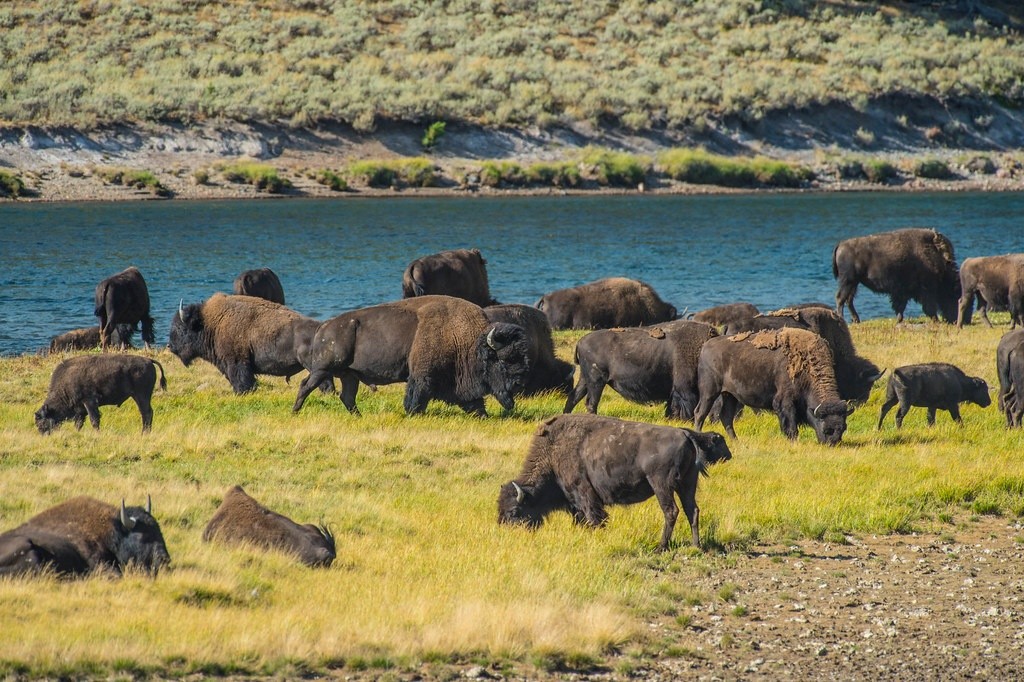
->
[47,320,142,354]
[0,494,172,579]
[872,362,992,432]
[34,354,167,439]
[495,412,732,554]
[94,266,155,353]
[834,227,1024,331]
[200,485,336,570]
[232,267,286,306]
[167,290,337,396]
[996,329,1024,428]
[293,249,888,446]
